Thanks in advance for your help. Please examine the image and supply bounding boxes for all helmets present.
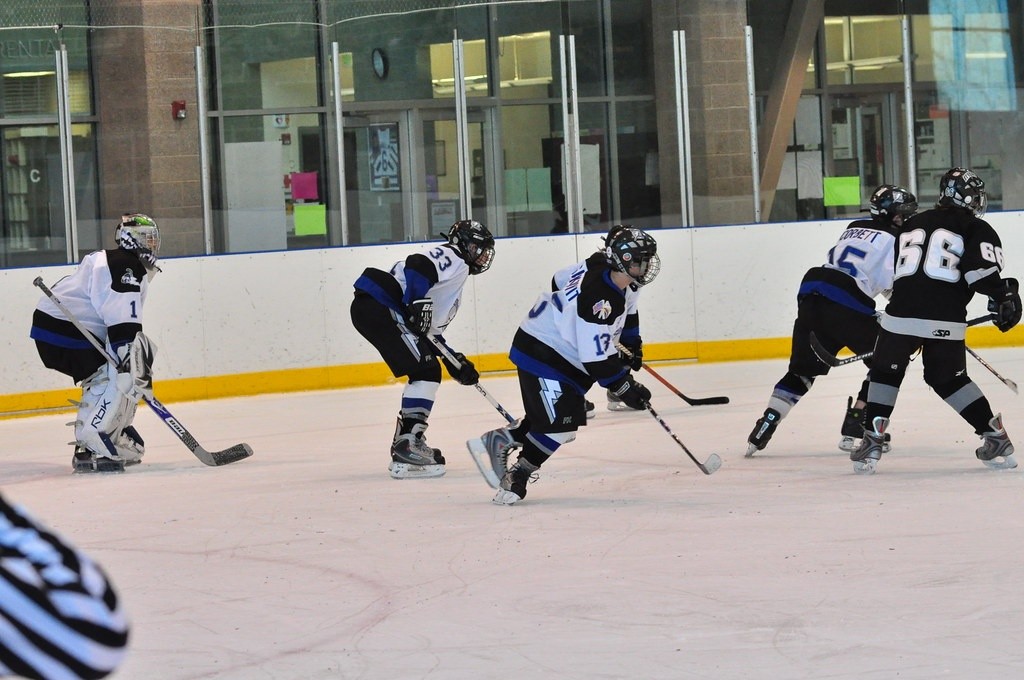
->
[869,184,918,227]
[938,167,987,220]
[600,223,661,292]
[114,213,161,265]
[440,220,496,274]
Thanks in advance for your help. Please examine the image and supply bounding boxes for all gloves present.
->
[441,351,480,386]
[987,277,1022,333]
[608,374,651,411]
[625,336,643,372]
[403,298,433,335]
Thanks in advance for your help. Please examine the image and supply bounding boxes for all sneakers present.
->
[70,446,127,474]
[583,398,596,419]
[389,421,447,480]
[849,416,890,475]
[975,412,1019,470]
[744,408,780,458]
[491,450,541,505]
[607,390,637,412]
[466,418,525,489]
[838,395,892,453]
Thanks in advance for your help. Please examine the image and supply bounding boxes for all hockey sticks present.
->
[646,403,723,475]
[426,333,521,431]
[33,275,254,467]
[615,343,730,406]
[807,314,998,368]
[961,344,1021,395]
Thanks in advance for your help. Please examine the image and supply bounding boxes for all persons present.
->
[746,184,917,450]
[482,223,651,499]
[0,488,133,680]
[850,167,1022,461]
[350,220,496,466]
[583,299,644,409]
[29,213,160,465]
